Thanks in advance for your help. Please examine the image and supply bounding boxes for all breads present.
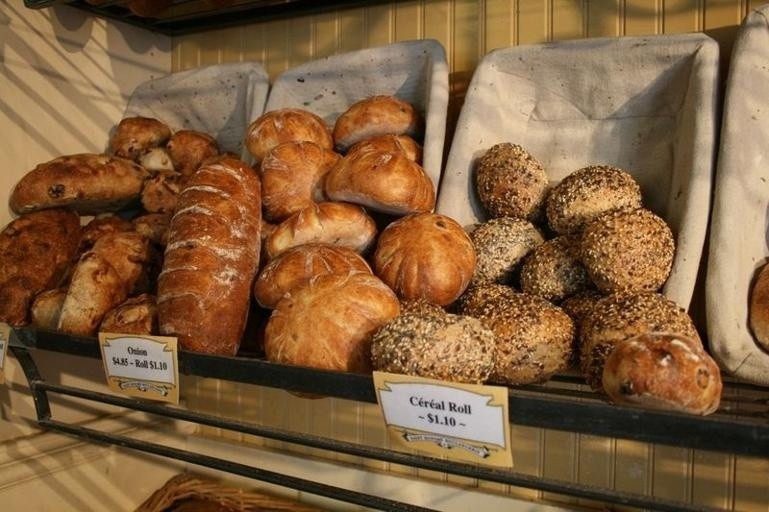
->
[360,137,725,414]
[748,263,769,354]
[0,117,279,350]
[245,89,475,371]
